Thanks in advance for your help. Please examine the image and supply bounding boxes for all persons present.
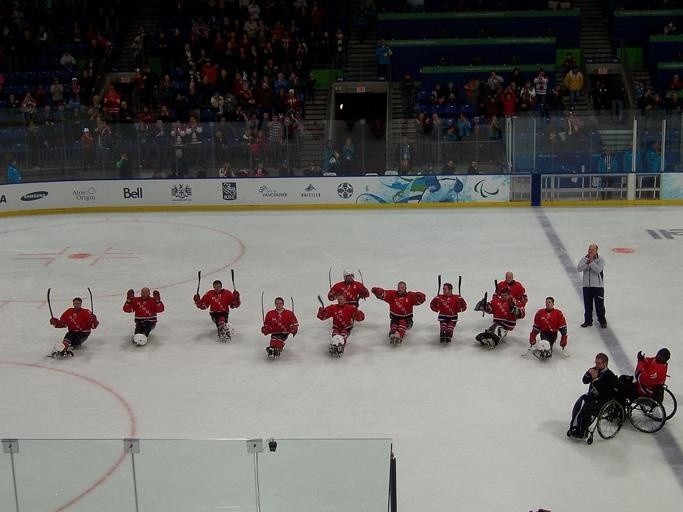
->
[371,281,426,344]
[193,280,241,342]
[1,1,683,184]
[328,268,370,309]
[261,297,299,360]
[317,292,365,358]
[429,283,467,343]
[50,298,99,359]
[123,287,164,346]
[474,245,671,439]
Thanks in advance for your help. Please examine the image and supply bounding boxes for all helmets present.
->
[51,341,65,355]
[343,269,354,280]
[133,333,148,346]
[536,339,551,351]
[329,334,345,346]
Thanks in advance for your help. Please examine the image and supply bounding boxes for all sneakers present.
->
[571,427,580,439]
[581,321,593,327]
[600,322,607,328]
[575,430,588,441]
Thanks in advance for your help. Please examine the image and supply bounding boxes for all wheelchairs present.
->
[566,392,624,444]
[626,372,677,434]
